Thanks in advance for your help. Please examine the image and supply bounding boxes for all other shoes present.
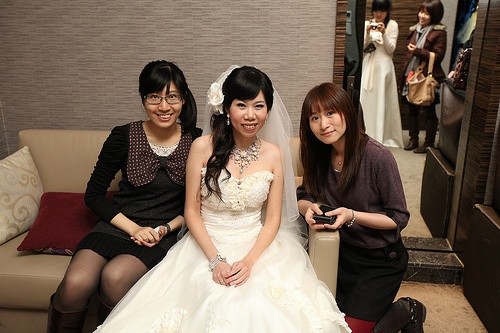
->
[405,138,420,150]
[414,142,433,153]
[398,297,427,333]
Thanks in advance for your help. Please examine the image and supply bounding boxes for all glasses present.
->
[143,93,183,104]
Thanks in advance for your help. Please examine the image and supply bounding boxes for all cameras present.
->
[370,23,378,30]
[314,205,338,224]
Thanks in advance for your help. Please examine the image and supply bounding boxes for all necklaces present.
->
[335,153,344,165]
[226,134,263,174]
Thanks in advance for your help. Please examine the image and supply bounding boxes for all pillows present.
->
[17,192,98,255]
[0,145,44,245]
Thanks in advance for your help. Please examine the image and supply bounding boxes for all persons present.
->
[296,82,427,333]
[362,0,405,148]
[47,60,204,333]
[93,65,353,333]
[402,0,447,153]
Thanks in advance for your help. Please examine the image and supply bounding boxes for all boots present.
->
[95,289,114,328]
[47,291,91,333]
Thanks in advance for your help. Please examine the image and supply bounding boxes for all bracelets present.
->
[346,211,356,227]
[163,223,171,233]
[208,254,226,272]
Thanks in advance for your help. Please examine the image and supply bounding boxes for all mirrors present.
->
[360,0,491,252]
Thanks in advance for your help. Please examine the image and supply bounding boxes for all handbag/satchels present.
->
[404,51,440,106]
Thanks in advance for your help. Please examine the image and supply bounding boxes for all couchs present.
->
[0,131,340,333]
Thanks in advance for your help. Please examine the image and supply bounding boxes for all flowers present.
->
[207,81,225,116]
